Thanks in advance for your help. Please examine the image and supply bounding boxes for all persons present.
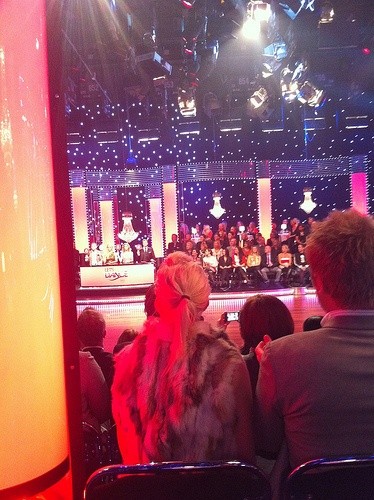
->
[112,250,254,479]
[254,209,374,471]
[78,294,324,500]
[82,215,316,292]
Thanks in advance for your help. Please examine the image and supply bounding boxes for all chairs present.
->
[81,454,374,500]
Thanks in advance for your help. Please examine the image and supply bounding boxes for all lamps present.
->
[209,192,227,219]
[117,211,140,244]
[177,1,334,118]
[300,187,318,214]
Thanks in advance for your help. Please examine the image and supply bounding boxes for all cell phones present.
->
[224,312,240,321]
